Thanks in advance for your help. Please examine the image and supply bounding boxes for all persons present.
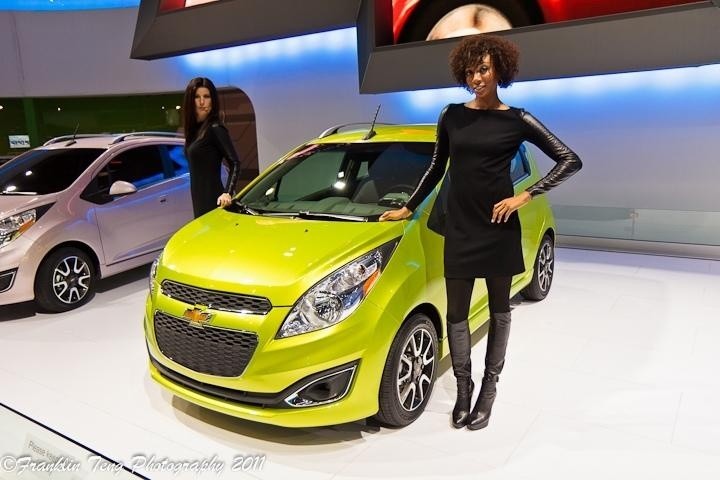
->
[183,78,243,220]
[379,36,585,431]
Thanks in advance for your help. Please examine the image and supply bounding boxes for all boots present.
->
[467,311,511,430]
[447,320,474,428]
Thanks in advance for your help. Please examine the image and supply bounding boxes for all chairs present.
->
[351,151,401,206]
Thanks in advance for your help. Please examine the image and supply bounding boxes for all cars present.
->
[1,120,235,314]
[141,103,556,428]
